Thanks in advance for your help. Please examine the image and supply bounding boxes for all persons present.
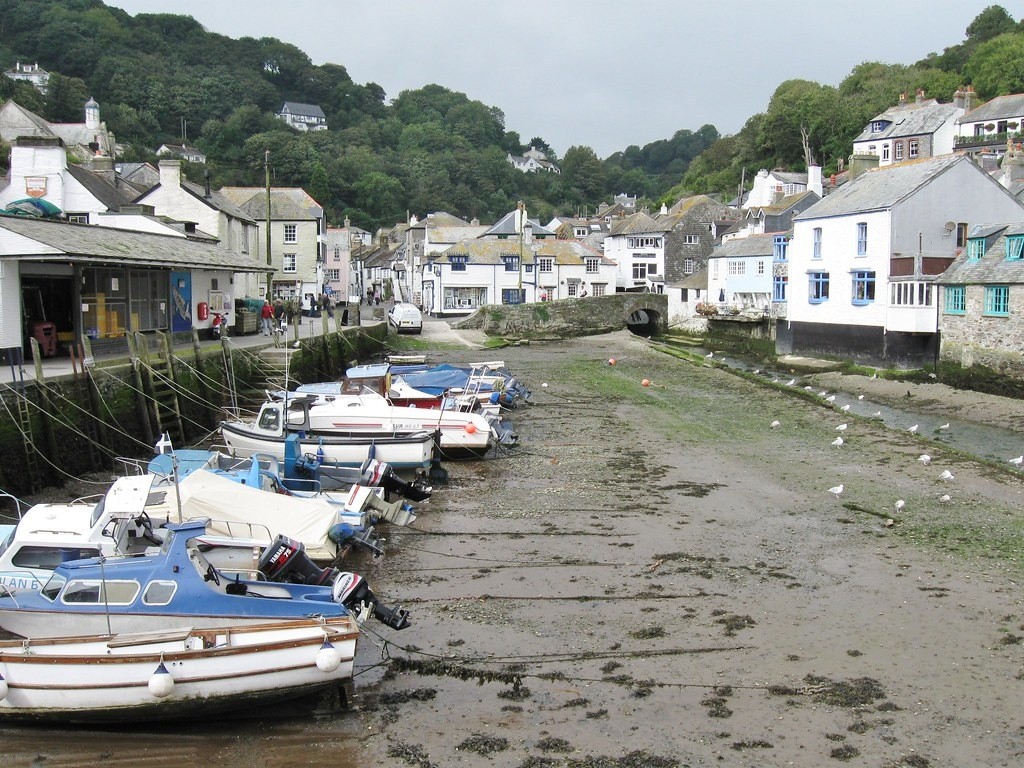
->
[260,298,285,336]
[367,287,380,306]
[308,293,333,318]
[646,283,656,294]
[539,285,547,302]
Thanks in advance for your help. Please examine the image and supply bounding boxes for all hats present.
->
[276,298,282,302]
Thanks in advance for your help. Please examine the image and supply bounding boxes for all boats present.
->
[272,394,492,459]
[146,445,433,522]
[0,474,414,632]
[0,520,358,659]
[294,356,531,420]
[219,404,442,467]
[0,617,362,723]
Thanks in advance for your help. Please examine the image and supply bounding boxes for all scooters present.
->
[209,307,230,339]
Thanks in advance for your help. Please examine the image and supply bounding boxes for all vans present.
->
[389,302,422,333]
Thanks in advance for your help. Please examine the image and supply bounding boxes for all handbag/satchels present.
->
[270,313,275,319]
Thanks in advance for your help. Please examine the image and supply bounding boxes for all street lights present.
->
[517,200,526,301]
[262,148,273,309]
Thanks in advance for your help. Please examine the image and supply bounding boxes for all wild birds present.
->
[827,483,845,501]
[937,467,957,486]
[767,418,783,430]
[868,373,878,383]
[705,351,714,360]
[857,393,867,402]
[871,409,882,421]
[1007,453,1023,469]
[937,493,953,508]
[829,422,849,449]
[752,368,851,413]
[915,452,933,466]
[905,420,952,437]
[719,356,727,363]
[893,497,906,514]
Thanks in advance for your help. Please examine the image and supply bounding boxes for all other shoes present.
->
[264,334,267,336]
[270,332,272,335]
